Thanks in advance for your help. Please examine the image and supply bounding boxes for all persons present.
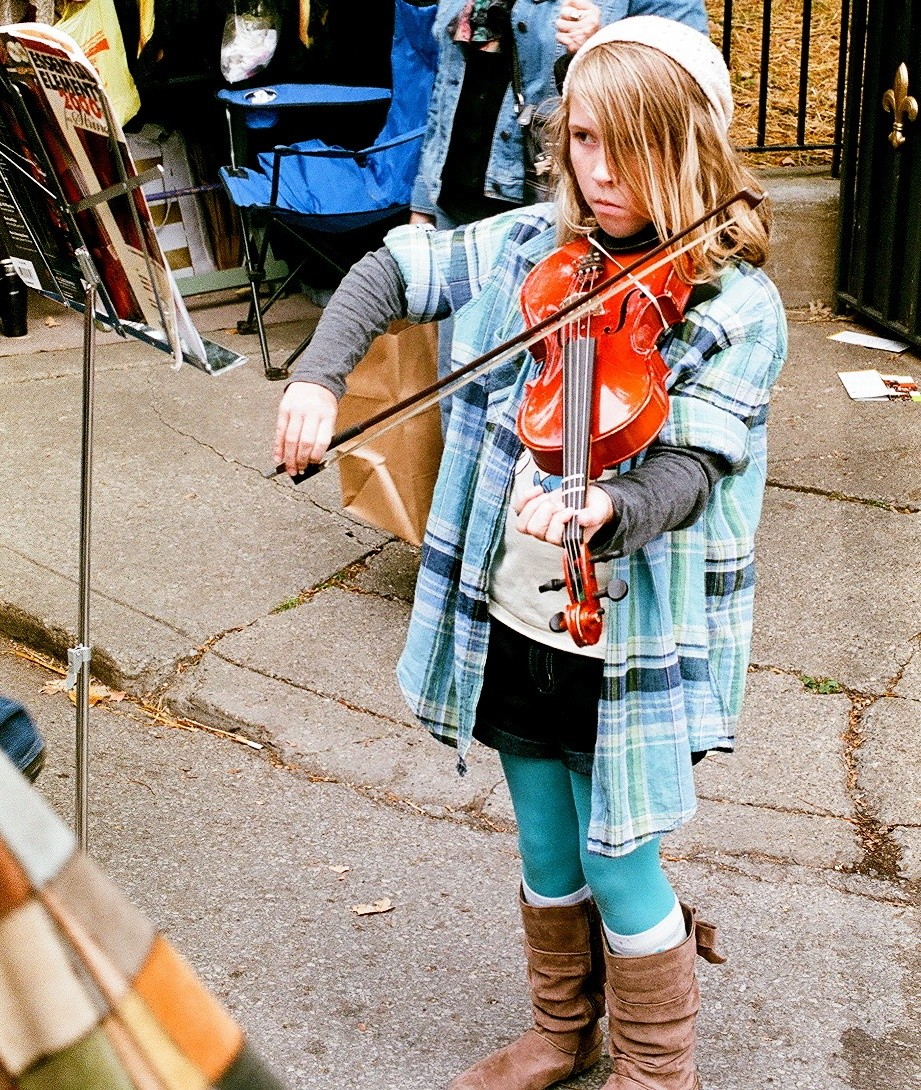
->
[410,0,707,454]
[275,17,789,1090]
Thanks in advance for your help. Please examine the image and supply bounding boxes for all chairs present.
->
[218,83,427,379]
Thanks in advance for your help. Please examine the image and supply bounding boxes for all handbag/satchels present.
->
[522,112,571,207]
[335,319,446,546]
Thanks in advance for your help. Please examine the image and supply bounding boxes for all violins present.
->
[515,233,698,648]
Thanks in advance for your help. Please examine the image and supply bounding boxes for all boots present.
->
[583,901,730,1087]
[448,884,604,1089]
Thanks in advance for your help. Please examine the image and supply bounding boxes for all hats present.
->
[563,14,733,139]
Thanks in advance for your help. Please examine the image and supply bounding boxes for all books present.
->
[0,24,208,371]
